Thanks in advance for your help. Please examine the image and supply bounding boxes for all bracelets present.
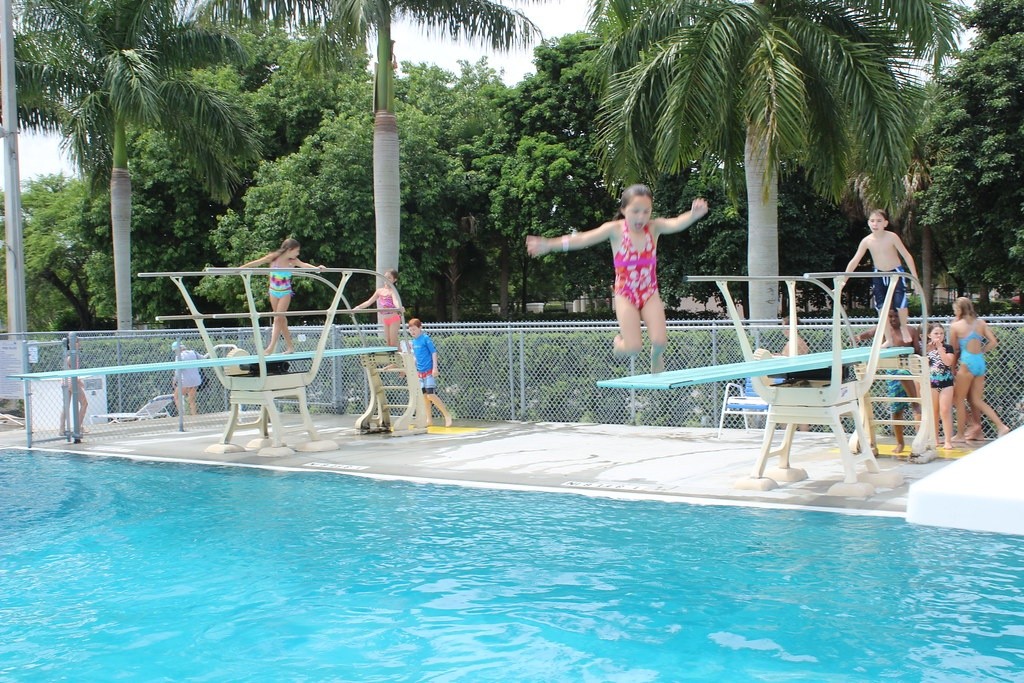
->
[562,235,569,252]
[842,282,846,286]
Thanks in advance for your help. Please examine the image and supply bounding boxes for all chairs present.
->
[719,378,784,434]
[90,395,174,426]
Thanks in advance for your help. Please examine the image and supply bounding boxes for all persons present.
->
[349,269,406,378]
[832,210,920,348]
[917,322,981,450]
[941,297,1009,444]
[408,319,452,427]
[240,238,327,356]
[526,185,708,374]
[849,310,922,453]
[780,317,809,433]
[171,342,210,416]
[59,336,90,436]
